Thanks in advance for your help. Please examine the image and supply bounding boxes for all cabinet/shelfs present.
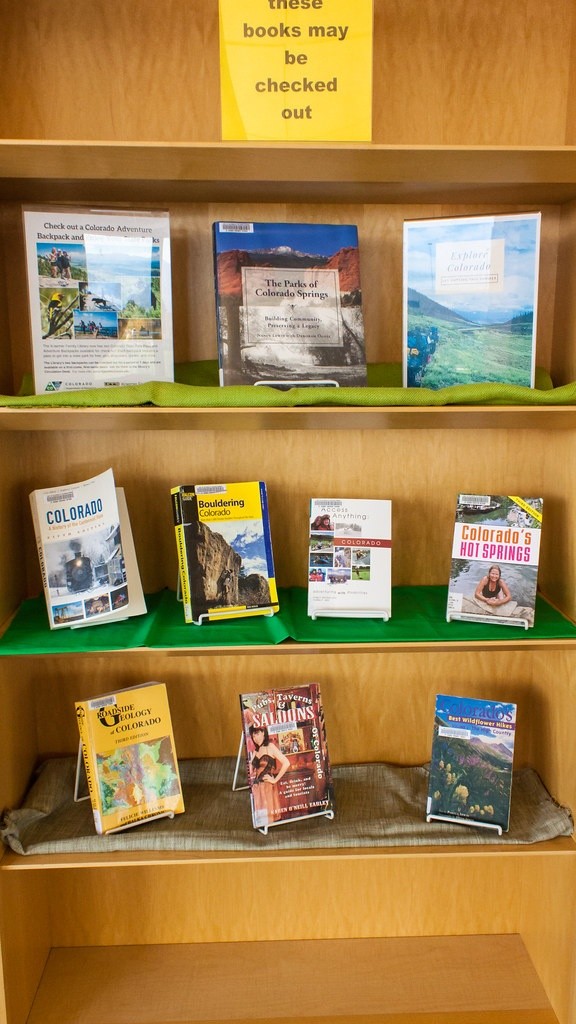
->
[0,0,571,1024]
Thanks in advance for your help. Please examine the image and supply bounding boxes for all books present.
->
[402,209,541,390]
[28,467,147,630]
[211,220,366,387]
[446,493,544,628]
[75,681,187,833]
[22,203,174,395]
[169,482,280,623]
[238,682,335,828]
[426,694,517,833]
[307,496,393,618]
